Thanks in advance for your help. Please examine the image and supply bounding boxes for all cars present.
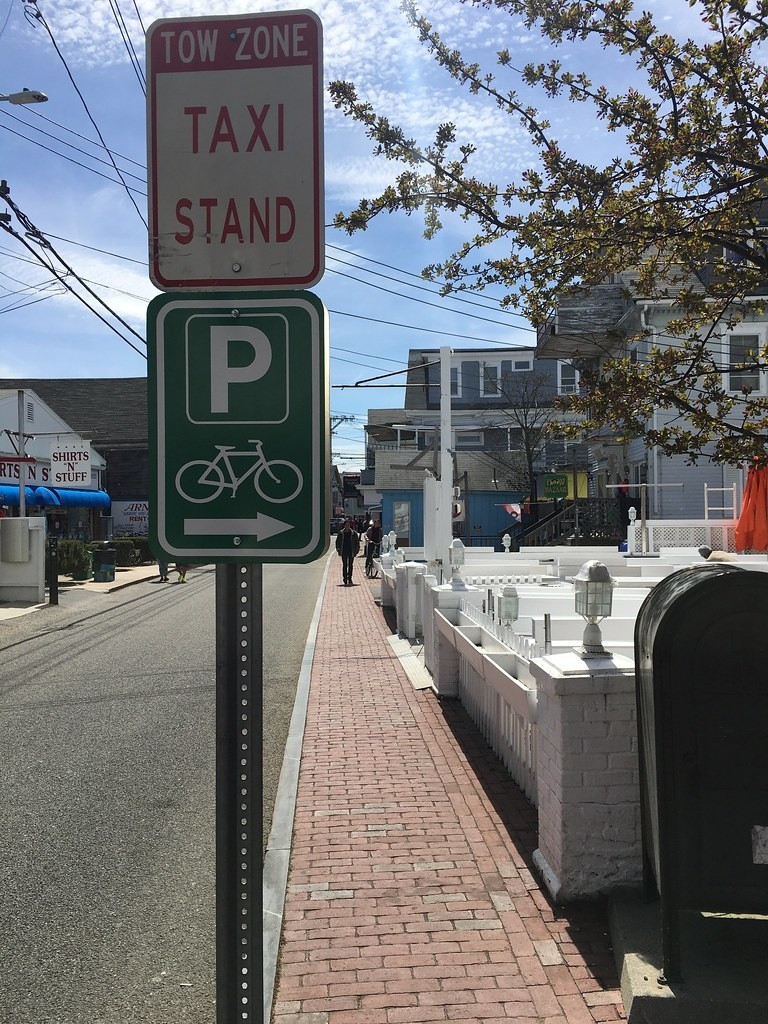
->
[330,517,345,534]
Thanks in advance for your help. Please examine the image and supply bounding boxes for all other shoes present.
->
[165,577,169,581]
[343,577,348,584]
[160,578,163,582]
[348,580,353,585]
[178,574,187,582]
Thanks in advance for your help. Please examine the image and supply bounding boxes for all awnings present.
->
[0,484,111,508]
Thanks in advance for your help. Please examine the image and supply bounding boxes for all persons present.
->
[175,563,190,583]
[363,519,382,573]
[159,562,169,581]
[697,545,740,562]
[346,516,382,541]
[335,520,360,587]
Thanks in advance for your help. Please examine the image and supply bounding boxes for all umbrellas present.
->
[734,450,768,552]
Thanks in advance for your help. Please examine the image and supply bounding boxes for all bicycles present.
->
[365,542,383,578]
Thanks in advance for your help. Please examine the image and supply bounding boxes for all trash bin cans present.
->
[93,540,116,582]
